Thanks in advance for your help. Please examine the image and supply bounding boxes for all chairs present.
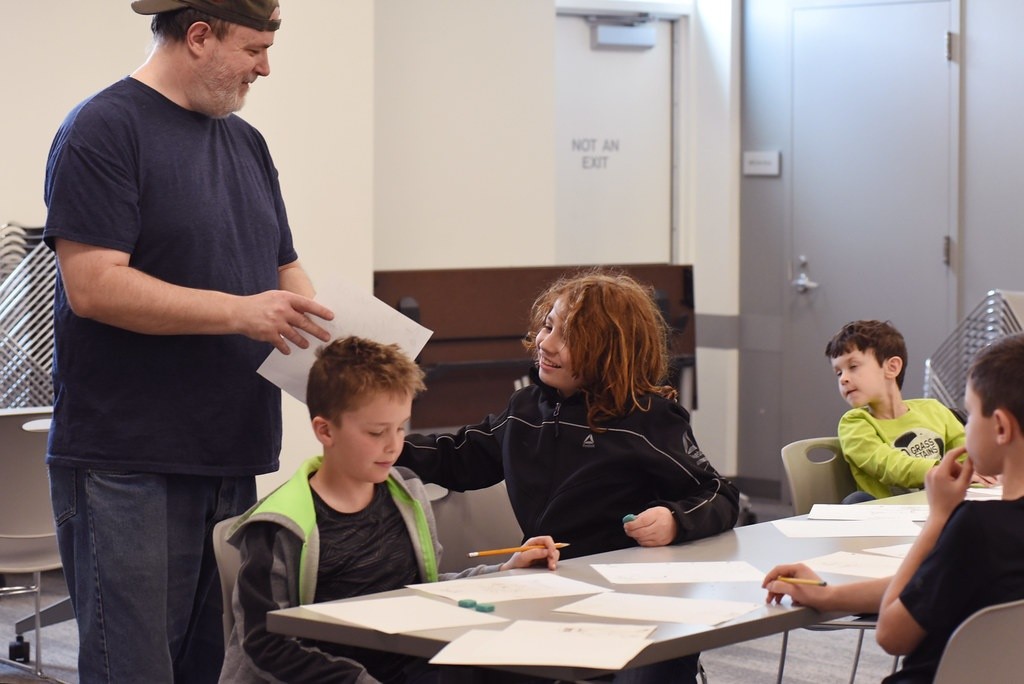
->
[924,289,1024,421]
[776,436,904,684]
[0,221,57,406]
[929,598,1024,684]
[0,406,76,684]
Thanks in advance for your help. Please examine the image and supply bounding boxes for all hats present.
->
[131,0,281,30]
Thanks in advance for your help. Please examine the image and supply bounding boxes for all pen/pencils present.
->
[467,542,571,558]
[776,576,828,587]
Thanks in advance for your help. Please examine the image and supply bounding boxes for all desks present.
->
[265,481,1004,684]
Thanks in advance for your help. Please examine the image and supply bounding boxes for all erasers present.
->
[623,514,635,524]
[458,598,475,608]
[475,603,495,612]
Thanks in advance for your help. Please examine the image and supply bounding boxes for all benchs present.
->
[374,263,698,429]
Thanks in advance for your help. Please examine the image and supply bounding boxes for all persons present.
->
[44,0,335,684]
[826,319,1001,505]
[217,335,560,684]
[761,331,1024,683]
[393,271,740,684]
